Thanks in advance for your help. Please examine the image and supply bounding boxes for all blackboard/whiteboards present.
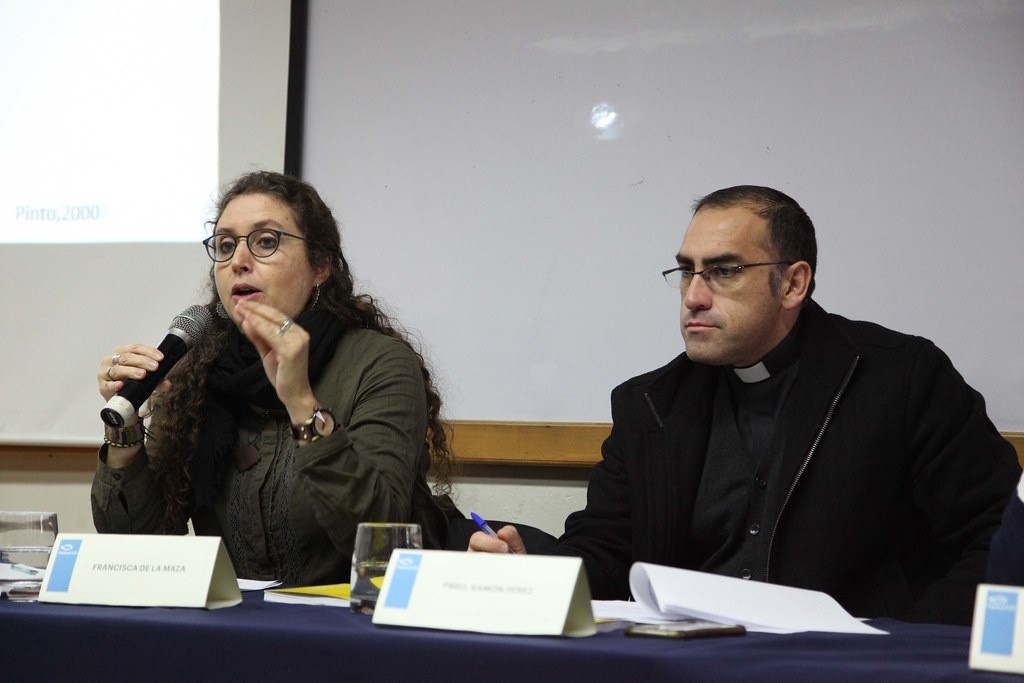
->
[303,1,1024,435]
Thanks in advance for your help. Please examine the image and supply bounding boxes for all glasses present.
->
[202,228,316,263]
[662,261,794,292]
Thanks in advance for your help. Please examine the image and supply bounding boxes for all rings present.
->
[113,353,122,365]
[276,319,293,334]
[106,368,116,381]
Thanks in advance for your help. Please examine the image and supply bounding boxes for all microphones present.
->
[100,304,213,428]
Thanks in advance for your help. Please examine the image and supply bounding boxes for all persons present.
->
[467,186,1024,629]
[90,169,466,590]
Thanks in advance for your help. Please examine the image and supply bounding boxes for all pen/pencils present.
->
[470,511,515,553]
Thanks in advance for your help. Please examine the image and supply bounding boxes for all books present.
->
[260,578,353,609]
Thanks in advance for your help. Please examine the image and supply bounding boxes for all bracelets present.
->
[98,415,143,448]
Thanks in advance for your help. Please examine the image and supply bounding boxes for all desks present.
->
[1,562,1024,683]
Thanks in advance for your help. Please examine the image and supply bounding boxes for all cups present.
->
[0,511,59,590]
[348,522,424,619]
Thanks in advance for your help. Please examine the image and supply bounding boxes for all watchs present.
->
[287,406,338,442]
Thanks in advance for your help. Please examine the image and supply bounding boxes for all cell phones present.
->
[624,621,745,640]
